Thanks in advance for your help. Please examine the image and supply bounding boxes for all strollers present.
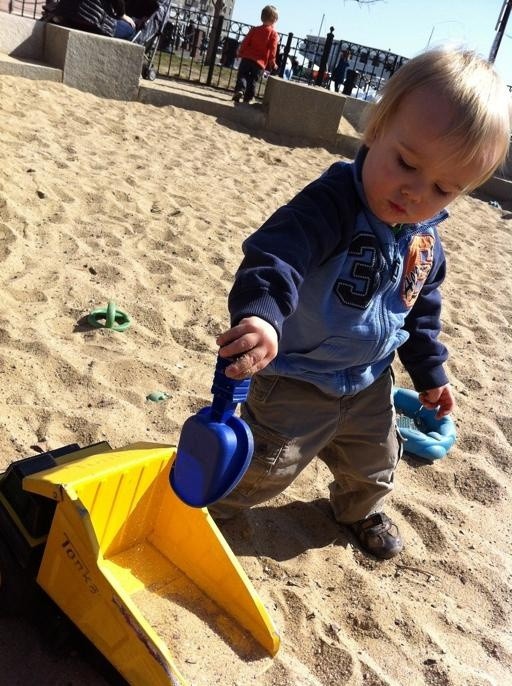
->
[131,0,171,82]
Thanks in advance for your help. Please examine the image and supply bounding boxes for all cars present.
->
[291,48,331,87]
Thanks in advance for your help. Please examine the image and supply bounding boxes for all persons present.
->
[333,49,350,90]
[231,4,280,103]
[208,45,512,561]
[52,0,137,42]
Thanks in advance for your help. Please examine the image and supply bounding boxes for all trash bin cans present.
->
[342,69,361,96]
[220,37,240,69]
[157,22,175,54]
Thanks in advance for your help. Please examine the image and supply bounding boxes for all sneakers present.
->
[327,498,403,559]
